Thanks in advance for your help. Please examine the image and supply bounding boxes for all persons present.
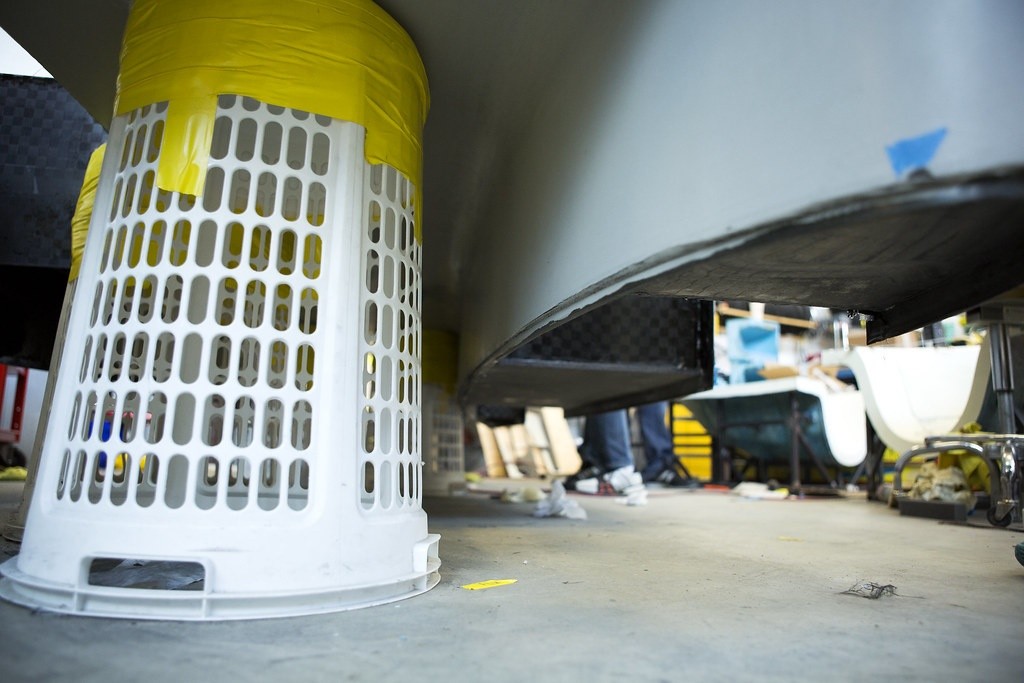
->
[573,402,686,494]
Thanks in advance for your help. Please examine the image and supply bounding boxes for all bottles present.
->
[727,302,779,384]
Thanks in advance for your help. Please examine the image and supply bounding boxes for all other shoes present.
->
[569,465,642,495]
[645,469,705,490]
